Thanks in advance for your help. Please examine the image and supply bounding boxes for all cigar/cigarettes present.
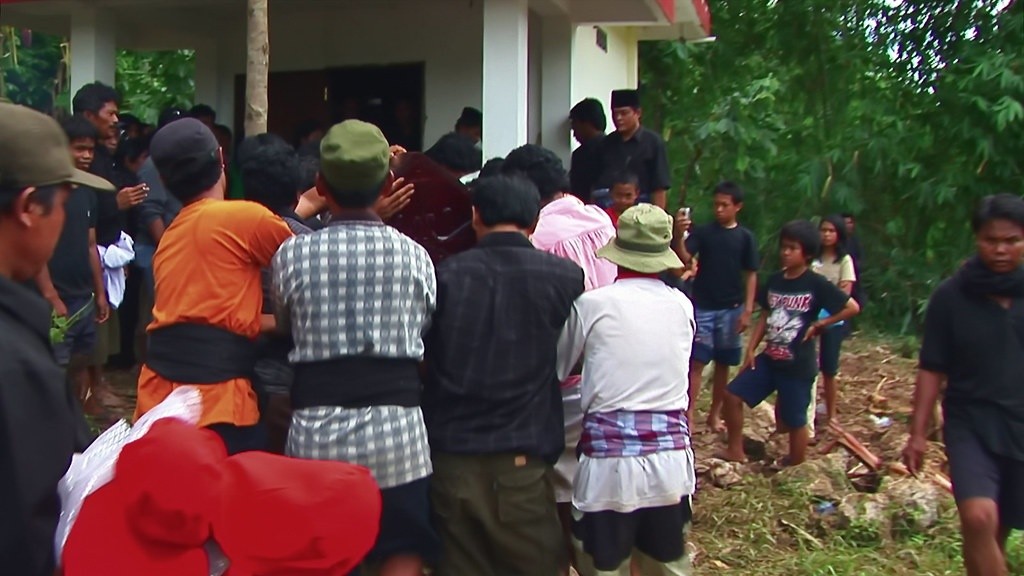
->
[142,187,146,190]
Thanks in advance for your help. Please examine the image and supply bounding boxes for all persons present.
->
[0,81,861,576]
[902,195,1024,576]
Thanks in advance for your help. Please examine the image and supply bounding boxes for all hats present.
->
[611,90,640,108]
[595,203,685,273]
[319,119,389,205]
[1,97,115,191]
[150,118,219,175]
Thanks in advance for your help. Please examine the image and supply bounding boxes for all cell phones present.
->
[678,207,690,220]
